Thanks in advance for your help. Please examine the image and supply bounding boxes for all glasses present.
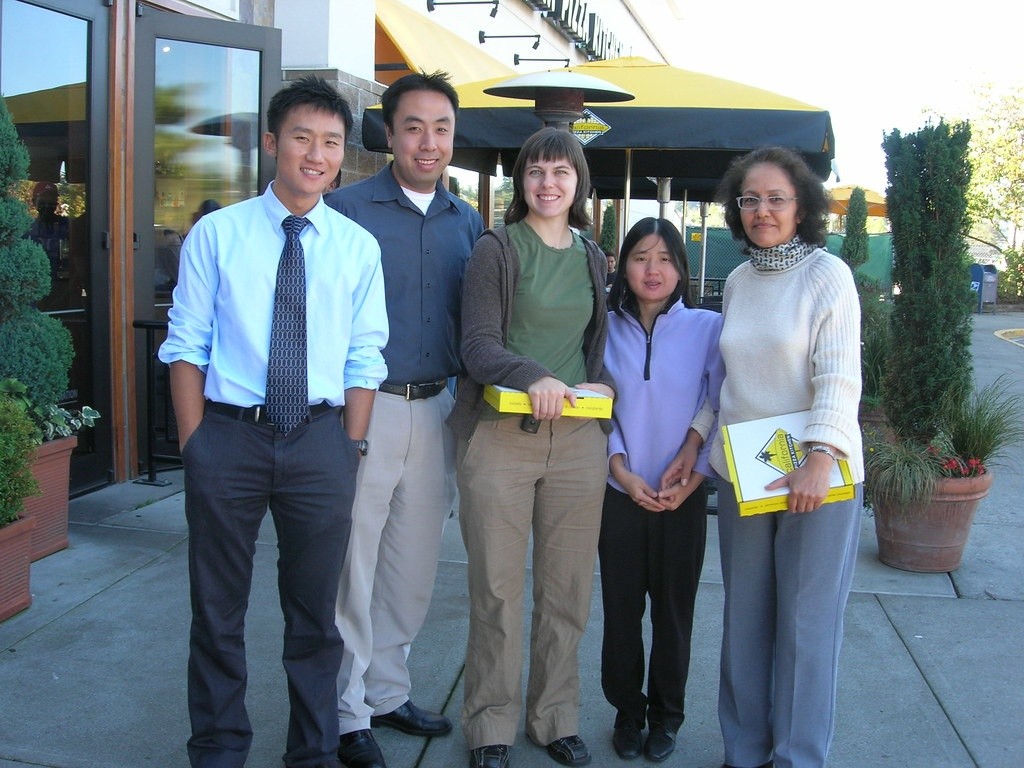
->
[734,195,802,211]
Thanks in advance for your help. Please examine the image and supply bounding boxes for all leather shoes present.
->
[337,727,387,768]
[644,725,678,761]
[614,716,642,759]
[370,698,453,736]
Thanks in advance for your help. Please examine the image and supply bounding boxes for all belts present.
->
[205,398,332,428]
[379,380,449,400]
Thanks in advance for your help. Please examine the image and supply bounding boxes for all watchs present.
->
[353,440,367,455]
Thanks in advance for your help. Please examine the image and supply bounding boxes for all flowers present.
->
[864,381,1024,520]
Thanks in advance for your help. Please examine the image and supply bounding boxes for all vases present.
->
[866,467,993,573]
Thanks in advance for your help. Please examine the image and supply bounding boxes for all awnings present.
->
[376,0,516,90]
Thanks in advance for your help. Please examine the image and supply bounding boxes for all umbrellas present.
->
[362,55,836,304]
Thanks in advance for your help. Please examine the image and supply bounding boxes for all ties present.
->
[266,216,312,435]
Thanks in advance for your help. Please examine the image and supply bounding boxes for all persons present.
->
[25,183,70,266]
[605,252,617,285]
[157,75,389,767]
[660,147,866,768]
[441,126,618,768]
[598,218,727,761]
[325,70,489,768]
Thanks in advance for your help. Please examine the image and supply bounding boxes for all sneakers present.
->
[471,745,509,768]
[548,732,592,767]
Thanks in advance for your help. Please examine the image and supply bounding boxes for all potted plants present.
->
[0,396,40,620]
[859,338,894,443]
[0,91,101,562]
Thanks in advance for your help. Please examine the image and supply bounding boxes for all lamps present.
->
[427,0,500,18]
[479,31,540,50]
[514,54,570,67]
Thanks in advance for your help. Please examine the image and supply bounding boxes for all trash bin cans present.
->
[969,263,1001,314]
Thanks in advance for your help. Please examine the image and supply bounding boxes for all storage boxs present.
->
[722,411,855,517]
[484,383,612,420]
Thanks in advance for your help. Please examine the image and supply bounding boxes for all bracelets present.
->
[807,446,835,460]
